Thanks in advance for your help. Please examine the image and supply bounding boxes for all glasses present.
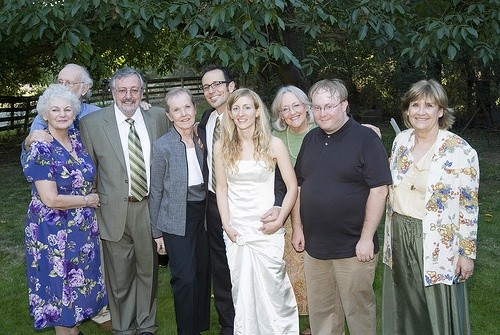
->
[310,100,344,112]
[201,81,230,90]
[58,80,85,88]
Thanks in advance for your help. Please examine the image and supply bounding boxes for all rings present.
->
[470,273,473,275]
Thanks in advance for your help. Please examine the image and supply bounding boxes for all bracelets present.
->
[84,194,89,207]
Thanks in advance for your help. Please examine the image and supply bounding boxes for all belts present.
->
[128,195,147,202]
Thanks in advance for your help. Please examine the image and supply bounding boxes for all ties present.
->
[125,117,149,202]
[212,116,221,192]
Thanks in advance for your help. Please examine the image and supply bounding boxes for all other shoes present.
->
[100,321,112,328]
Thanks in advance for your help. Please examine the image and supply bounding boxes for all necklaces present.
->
[67,135,74,154]
[287,121,309,159]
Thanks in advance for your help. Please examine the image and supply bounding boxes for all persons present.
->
[213,87,300,335]
[147,87,211,335]
[196,64,287,335]
[289,78,393,335]
[22,83,110,335]
[360,79,480,335]
[20,63,112,328]
[269,84,320,335]
[79,66,203,335]
